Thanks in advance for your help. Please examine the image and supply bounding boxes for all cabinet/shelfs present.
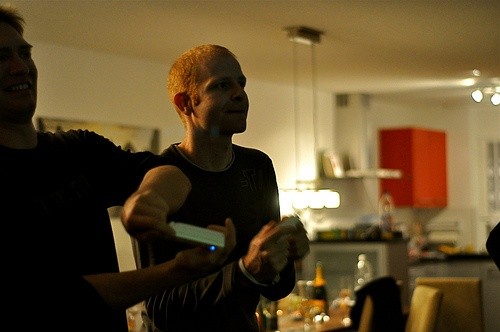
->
[379,127,447,208]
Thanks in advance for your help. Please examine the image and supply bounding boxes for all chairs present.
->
[348,275,482,332]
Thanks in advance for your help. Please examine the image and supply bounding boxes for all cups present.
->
[298,281,312,322]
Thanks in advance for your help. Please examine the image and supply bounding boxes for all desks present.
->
[298,234,408,311]
[274,312,350,332]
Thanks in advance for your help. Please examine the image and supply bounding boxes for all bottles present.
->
[357,254,373,287]
[312,260,329,317]
[379,189,394,238]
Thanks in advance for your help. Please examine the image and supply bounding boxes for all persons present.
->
[132,44,309,332]
[0,9,236,332]
